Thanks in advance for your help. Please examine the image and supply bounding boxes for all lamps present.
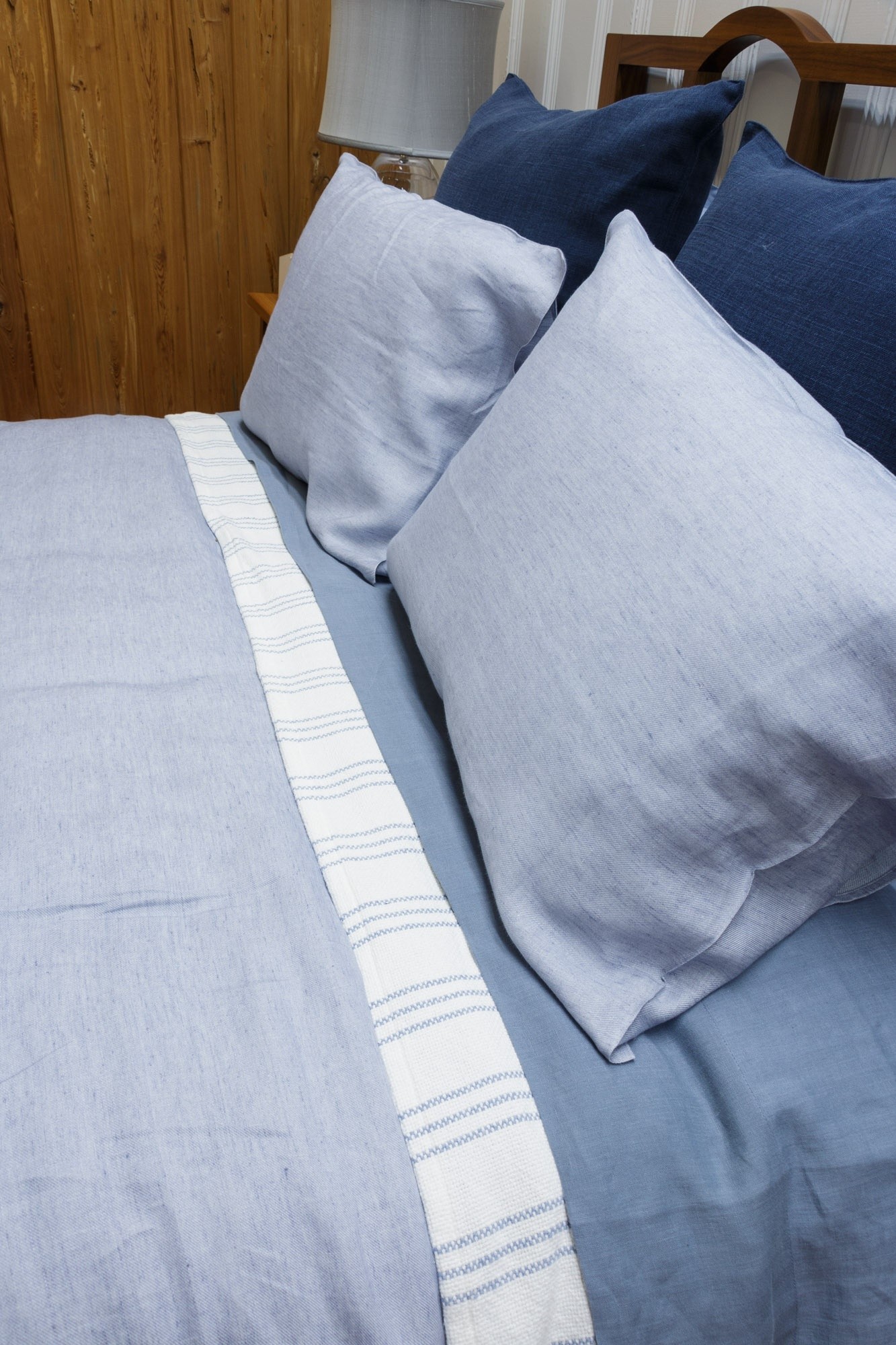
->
[317,0,506,203]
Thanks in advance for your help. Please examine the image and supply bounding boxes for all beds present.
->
[0,4,896,1345]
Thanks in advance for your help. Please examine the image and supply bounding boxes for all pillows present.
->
[676,119,896,472]
[438,71,747,310]
[377,210,896,1074]
[240,152,572,591]
